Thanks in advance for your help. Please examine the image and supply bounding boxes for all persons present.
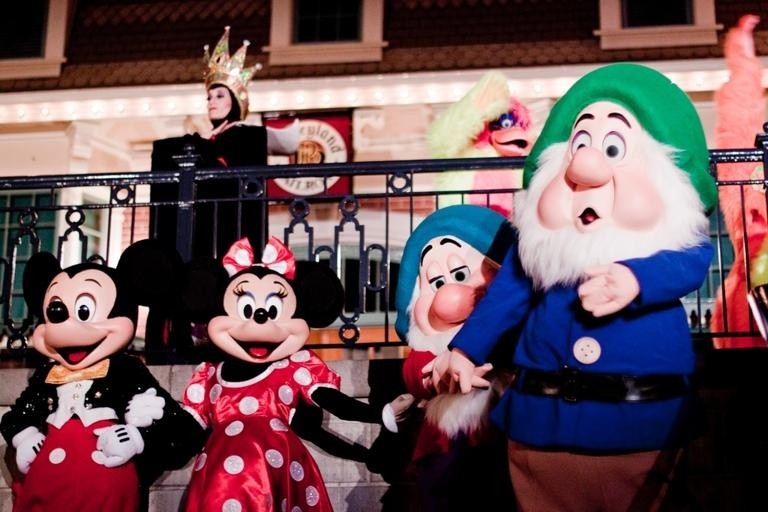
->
[146,84,268,365]
[380,205,518,512]
[422,59,718,512]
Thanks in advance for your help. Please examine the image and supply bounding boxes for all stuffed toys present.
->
[0,239,205,512]
[124,235,415,512]
[428,71,536,220]
[709,13,768,350]
